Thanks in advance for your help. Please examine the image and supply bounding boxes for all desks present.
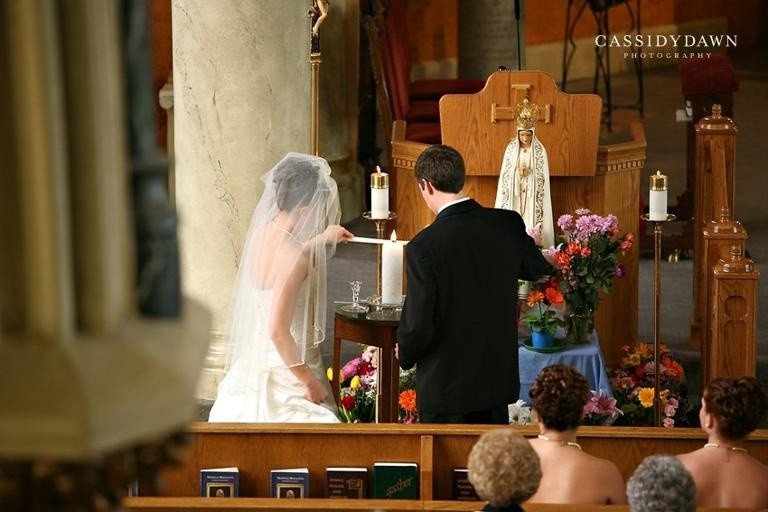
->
[330,301,407,425]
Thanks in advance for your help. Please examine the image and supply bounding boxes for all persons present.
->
[207,152,353,426]
[466,429,542,512]
[493,98,555,251]
[626,454,697,511]
[674,376,768,508]
[392,144,546,426]
[524,362,628,505]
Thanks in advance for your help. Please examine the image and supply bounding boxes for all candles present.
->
[369,166,389,220]
[380,229,404,305]
[649,170,668,220]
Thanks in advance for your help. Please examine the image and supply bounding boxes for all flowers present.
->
[506,202,689,429]
[325,342,422,424]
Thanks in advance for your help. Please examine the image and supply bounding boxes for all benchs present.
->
[114,420,766,512]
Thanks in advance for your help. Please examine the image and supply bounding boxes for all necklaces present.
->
[703,443,748,454]
[271,219,304,245]
[537,435,582,451]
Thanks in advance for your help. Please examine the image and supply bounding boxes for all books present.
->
[199,467,239,497]
[325,465,369,500]
[451,466,484,502]
[371,461,419,500]
[270,467,309,499]
